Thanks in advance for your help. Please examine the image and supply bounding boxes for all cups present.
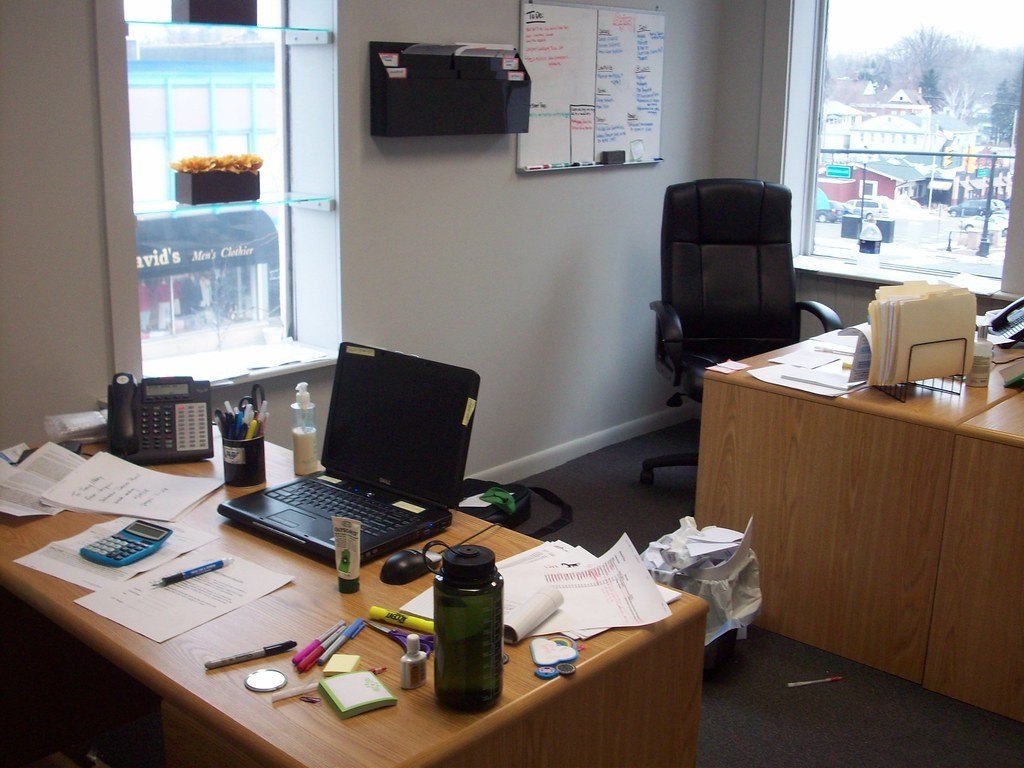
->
[966,339,993,387]
[222,435,266,487]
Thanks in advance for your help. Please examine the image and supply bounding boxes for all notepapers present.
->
[318,667,398,719]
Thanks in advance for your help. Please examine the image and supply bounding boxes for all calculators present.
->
[80,519,175,569]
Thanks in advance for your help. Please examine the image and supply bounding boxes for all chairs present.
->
[640,177,844,496]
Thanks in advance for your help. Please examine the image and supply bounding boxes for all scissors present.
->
[364,620,436,658]
[238,385,264,413]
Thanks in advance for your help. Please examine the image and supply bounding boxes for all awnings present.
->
[927,179,952,191]
[961,176,1011,191]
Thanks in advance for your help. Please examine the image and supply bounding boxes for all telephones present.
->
[988,296,1024,349]
[107,372,213,464]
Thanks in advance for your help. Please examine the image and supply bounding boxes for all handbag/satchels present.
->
[448,478,532,530]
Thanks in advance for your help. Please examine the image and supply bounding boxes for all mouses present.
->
[380,549,443,585]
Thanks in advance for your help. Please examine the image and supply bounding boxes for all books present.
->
[398,569,565,645]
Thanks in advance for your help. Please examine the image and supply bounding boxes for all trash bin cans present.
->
[641,527,756,674]
[978,239,990,256]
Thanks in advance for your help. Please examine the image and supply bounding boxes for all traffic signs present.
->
[976,169,988,177]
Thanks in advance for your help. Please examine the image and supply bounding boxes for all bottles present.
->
[401,634,427,689]
[423,540,503,713]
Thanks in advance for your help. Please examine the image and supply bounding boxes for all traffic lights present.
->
[943,147,954,166]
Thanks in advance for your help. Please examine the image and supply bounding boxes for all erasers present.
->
[322,653,360,677]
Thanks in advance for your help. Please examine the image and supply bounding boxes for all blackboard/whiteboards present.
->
[516,0,666,175]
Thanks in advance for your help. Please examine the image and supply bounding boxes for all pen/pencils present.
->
[292,617,364,672]
[814,347,854,356]
[271,680,322,701]
[366,666,387,676]
[216,401,269,442]
[206,640,296,668]
[153,557,232,585]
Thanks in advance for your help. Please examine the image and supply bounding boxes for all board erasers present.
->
[603,151,626,164]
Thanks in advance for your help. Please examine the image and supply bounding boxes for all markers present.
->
[549,163,570,167]
[370,601,435,635]
[574,162,592,166]
[525,164,548,170]
[639,158,663,161]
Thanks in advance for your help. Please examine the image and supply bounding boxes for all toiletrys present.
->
[965,324,993,388]
[331,515,360,594]
[289,382,319,475]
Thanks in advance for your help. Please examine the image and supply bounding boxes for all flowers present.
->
[171,153,261,175]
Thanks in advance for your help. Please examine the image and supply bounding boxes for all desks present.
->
[0,426,709,768]
[924,391,1024,718]
[688,295,1012,686]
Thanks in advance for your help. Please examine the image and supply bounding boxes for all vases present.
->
[172,0,257,26]
[174,171,258,205]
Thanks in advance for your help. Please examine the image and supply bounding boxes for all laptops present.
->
[217,341,480,565]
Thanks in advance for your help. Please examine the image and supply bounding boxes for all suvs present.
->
[844,200,889,220]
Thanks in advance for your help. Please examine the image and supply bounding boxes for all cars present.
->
[816,200,845,224]
[958,213,1008,238]
[947,199,1007,217]
[142,339,167,362]
